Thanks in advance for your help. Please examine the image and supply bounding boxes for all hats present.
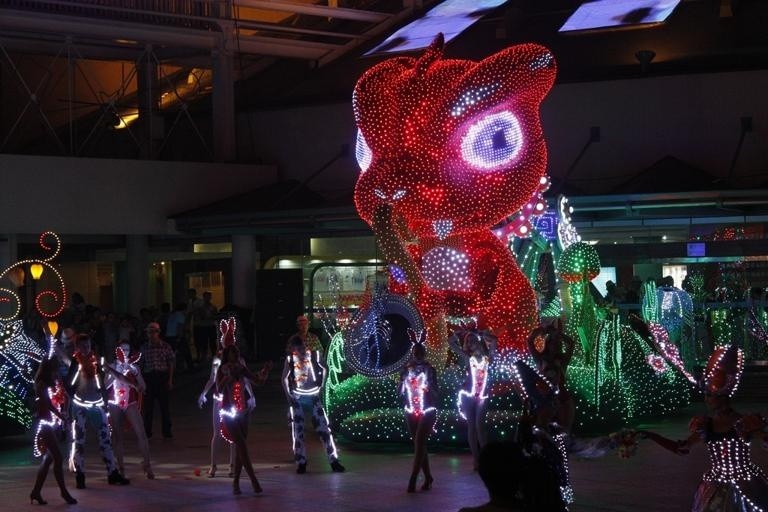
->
[296,315,309,325]
[143,323,161,333]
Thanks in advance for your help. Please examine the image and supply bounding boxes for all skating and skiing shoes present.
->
[75,479,87,490]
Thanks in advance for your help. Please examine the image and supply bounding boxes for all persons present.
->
[528,317,577,445]
[601,276,679,315]
[169,290,219,373]
[458,392,573,511]
[281,336,346,473]
[287,316,326,356]
[198,342,271,493]
[633,345,768,512]
[447,328,501,468]
[397,328,440,493]
[330,274,337,285]
[26,292,177,503]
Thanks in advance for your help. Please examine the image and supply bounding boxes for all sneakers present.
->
[60,490,77,504]
[330,459,347,473]
[106,469,130,485]
[295,461,308,474]
[407,477,433,493]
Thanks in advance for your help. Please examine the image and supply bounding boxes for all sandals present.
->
[206,466,264,495]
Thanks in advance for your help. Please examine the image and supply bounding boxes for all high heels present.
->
[30,490,49,507]
[142,461,155,479]
